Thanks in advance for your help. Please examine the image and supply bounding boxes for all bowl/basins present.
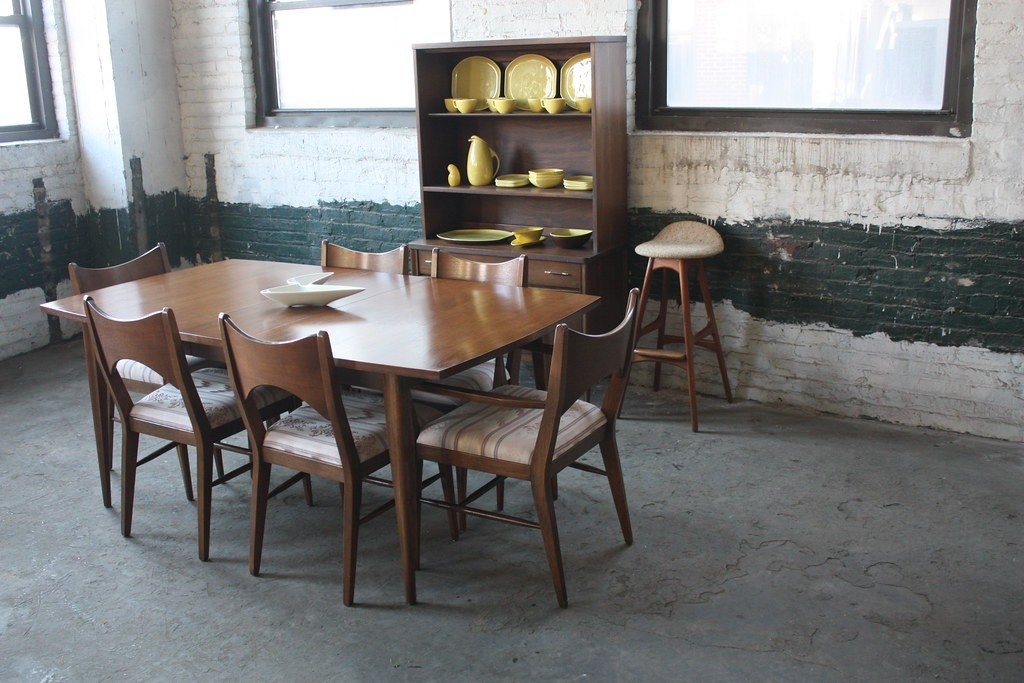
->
[259,271,366,308]
[512,226,543,243]
[528,168,563,188]
[550,229,592,248]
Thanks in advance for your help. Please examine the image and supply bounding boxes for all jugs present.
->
[466,135,500,186]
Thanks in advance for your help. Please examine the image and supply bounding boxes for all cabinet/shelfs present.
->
[409,35,630,368]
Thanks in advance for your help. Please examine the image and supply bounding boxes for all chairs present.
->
[67,238,639,608]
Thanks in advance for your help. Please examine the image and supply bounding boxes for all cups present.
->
[453,98,477,114]
[486,97,504,113]
[493,99,516,113]
[577,97,591,113]
[444,98,460,113]
[528,99,544,113]
[540,98,565,113]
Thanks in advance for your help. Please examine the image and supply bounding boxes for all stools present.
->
[613,219,732,434]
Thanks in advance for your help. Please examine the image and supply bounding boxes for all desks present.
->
[39,258,603,604]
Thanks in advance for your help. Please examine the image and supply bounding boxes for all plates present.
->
[495,174,530,187]
[563,175,593,190]
[437,229,514,242]
[504,53,557,110]
[559,53,591,109]
[511,235,546,247]
[452,56,502,111]
